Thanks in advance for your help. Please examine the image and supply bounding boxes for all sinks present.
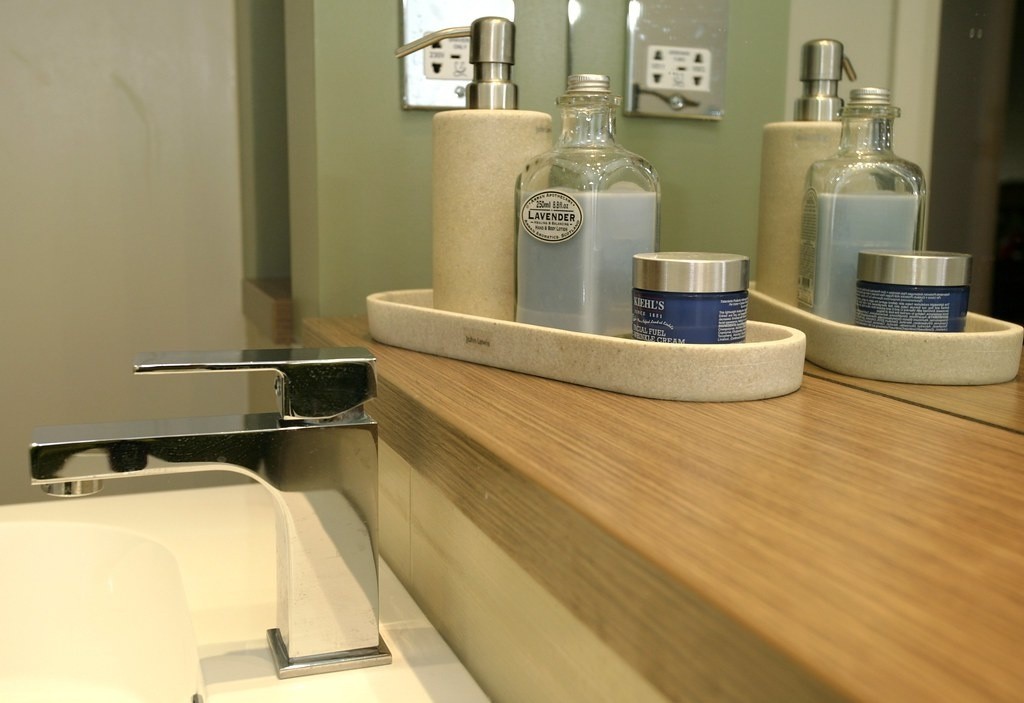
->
[2,518,208,702]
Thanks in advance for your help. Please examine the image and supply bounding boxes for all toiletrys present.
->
[757,38,973,332]
[395,16,748,345]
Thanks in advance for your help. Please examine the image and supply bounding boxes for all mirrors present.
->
[568,0,1024,435]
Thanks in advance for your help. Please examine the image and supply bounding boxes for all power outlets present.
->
[402,0,516,110]
[622,0,729,122]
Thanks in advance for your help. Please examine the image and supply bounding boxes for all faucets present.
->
[25,346,391,680]
